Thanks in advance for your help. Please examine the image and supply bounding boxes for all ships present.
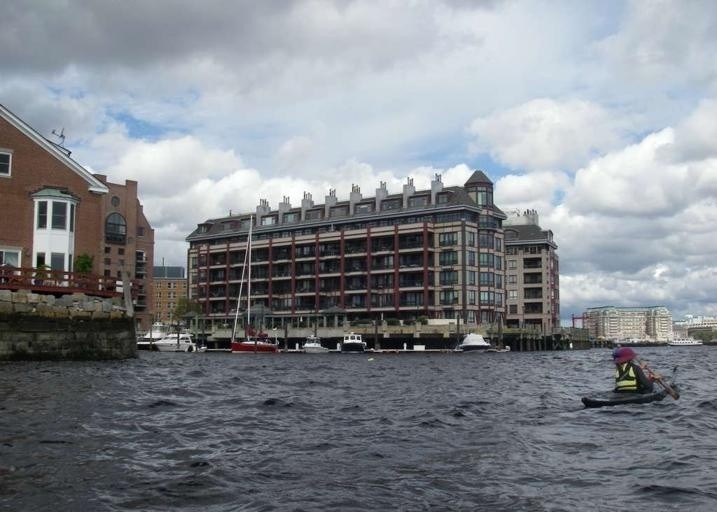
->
[666,337,703,345]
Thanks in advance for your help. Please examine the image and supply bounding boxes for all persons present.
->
[613,348,661,394]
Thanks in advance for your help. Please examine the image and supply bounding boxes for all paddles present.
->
[636,357,679,399]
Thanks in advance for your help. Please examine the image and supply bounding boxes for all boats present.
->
[340,331,366,353]
[135,320,184,349]
[301,335,328,353]
[151,332,209,353]
[458,333,492,352]
[579,379,675,409]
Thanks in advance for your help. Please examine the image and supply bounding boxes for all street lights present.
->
[371,319,377,344]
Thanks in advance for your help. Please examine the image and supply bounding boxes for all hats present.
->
[614,347,639,364]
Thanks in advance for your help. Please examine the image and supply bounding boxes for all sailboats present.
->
[229,213,282,354]
